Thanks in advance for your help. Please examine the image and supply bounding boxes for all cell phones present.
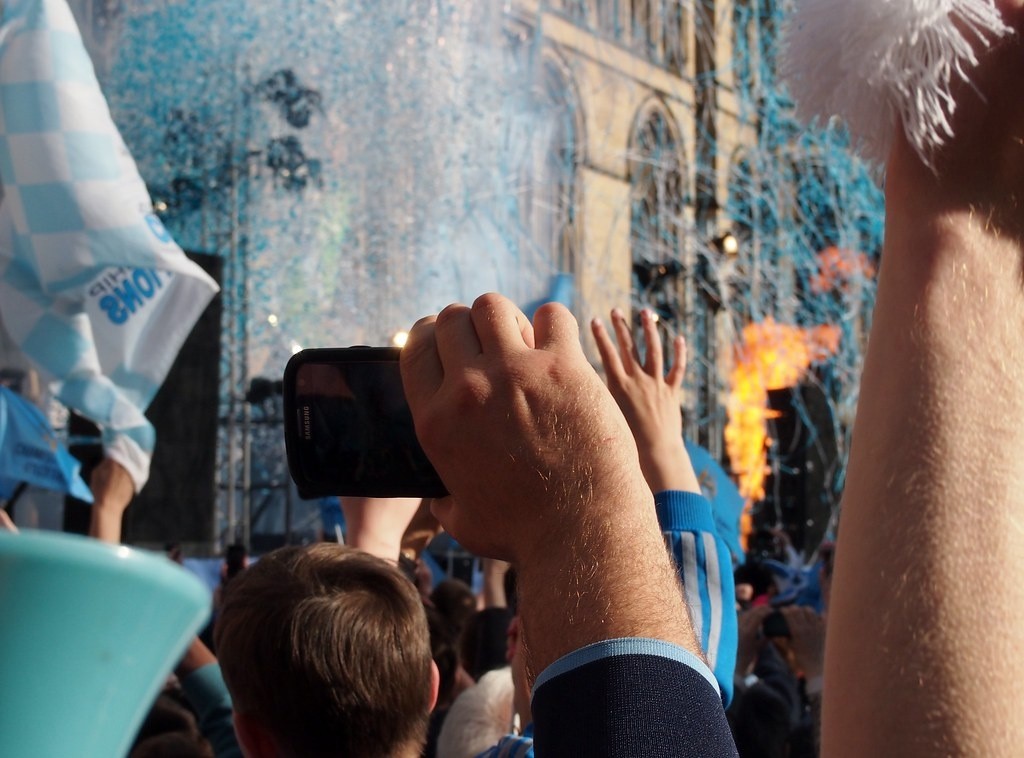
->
[762,611,792,640]
[283,344,449,500]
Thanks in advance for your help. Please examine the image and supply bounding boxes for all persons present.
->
[213,540,439,758]
[725,545,822,758]
[475,308,738,758]
[339,497,517,758]
[0,459,243,758]
[820,0,1024,758]
[399,294,738,758]
